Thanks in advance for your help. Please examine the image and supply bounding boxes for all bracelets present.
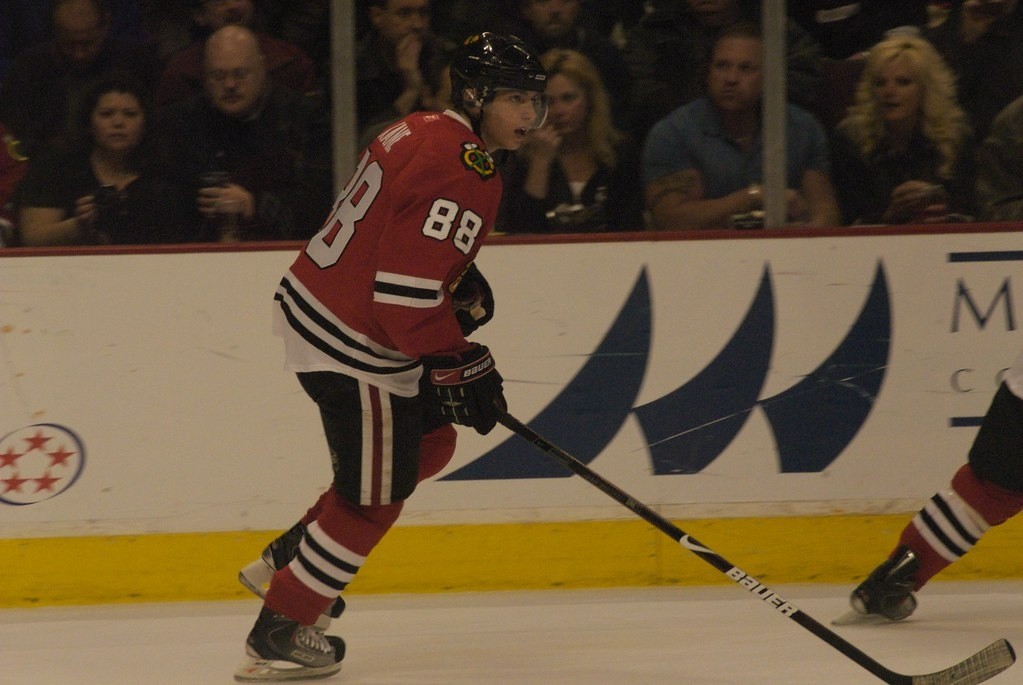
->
[745,184,763,209]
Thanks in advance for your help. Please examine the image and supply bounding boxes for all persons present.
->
[233,31,550,682]
[831,353,1023,630]
[1,1,1022,249]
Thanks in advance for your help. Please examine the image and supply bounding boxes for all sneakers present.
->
[234,602,347,681]
[832,542,918,626]
[238,520,346,630]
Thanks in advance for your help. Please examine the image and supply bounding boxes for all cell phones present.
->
[204,171,229,188]
[96,185,116,222]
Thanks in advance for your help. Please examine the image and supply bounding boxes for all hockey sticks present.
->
[488,400,1018,685]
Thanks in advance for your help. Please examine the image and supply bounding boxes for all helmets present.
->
[448,31,548,107]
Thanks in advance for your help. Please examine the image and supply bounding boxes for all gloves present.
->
[448,257,495,337]
[418,342,508,436]
